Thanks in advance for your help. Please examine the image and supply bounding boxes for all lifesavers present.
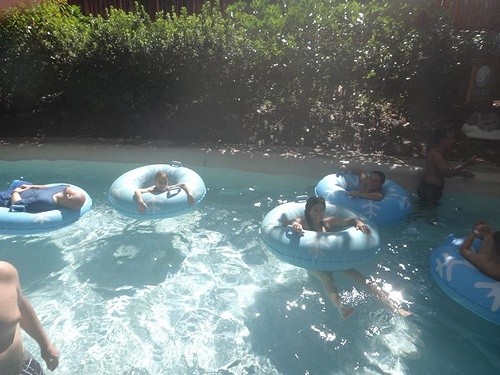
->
[429,233,500,326]
[0,183,93,236]
[314,172,411,224]
[107,161,206,220]
[259,195,380,271]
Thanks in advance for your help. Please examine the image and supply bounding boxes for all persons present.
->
[460,222,500,282]
[336,164,386,201]
[282,195,414,320]
[417,127,476,204]
[135,170,195,211]
[0,180,87,213]
[0,260,59,375]
